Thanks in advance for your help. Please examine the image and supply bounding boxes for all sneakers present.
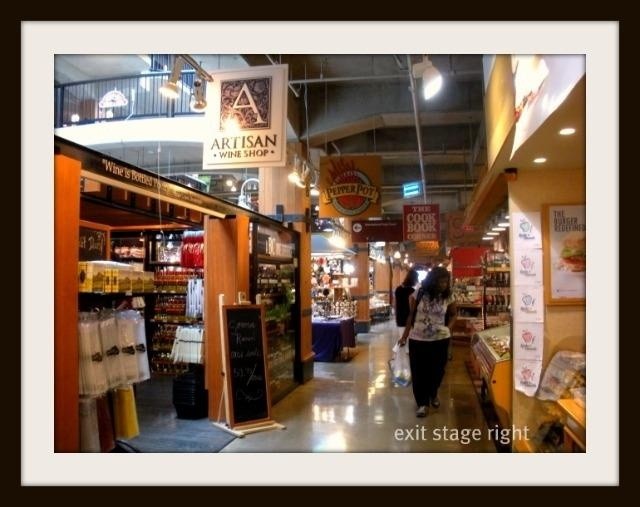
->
[416,399,440,415]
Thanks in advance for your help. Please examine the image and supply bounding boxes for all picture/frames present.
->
[542,203,586,306]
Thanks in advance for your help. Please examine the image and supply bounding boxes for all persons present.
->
[395,268,420,354]
[398,266,458,417]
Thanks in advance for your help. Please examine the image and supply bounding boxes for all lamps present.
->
[161,55,214,112]
[286,142,320,197]
[481,198,509,241]
[413,59,443,100]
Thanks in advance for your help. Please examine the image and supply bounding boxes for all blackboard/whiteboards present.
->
[219,302,272,429]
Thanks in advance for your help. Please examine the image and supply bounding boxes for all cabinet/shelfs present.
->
[310,255,347,305]
[250,254,298,404]
[148,240,203,373]
[471,324,512,445]
[483,249,512,328]
[448,303,483,359]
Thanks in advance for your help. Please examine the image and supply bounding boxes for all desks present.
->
[312,316,355,362]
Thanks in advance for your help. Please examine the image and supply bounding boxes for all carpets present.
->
[117,374,237,453]
